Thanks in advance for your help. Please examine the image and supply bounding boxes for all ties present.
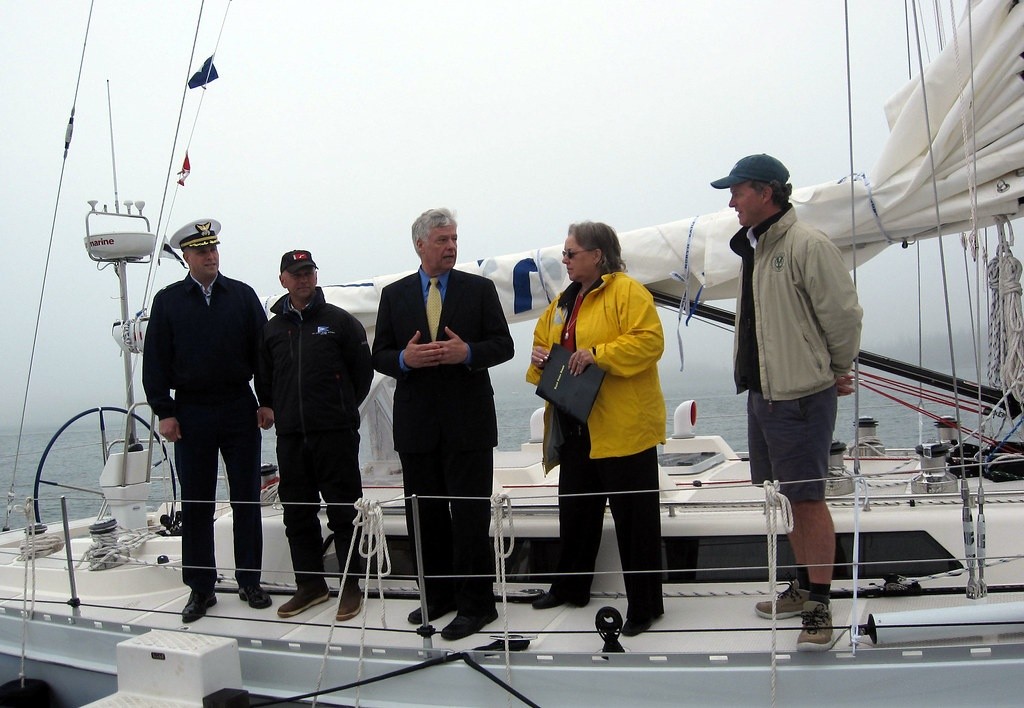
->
[425,277,443,341]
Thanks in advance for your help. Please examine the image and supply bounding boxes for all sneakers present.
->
[277,585,329,618]
[337,585,364,619]
[758,578,829,620]
[797,598,836,652]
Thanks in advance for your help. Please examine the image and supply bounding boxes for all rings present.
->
[577,361,582,364]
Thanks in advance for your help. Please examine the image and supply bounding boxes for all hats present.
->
[710,154,789,193]
[170,218,221,249]
[280,250,319,277]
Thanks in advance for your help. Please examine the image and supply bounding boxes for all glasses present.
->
[562,247,599,261]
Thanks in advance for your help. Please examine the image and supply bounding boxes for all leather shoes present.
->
[440,614,484,639]
[181,587,217,619]
[238,583,272,610]
[408,600,459,624]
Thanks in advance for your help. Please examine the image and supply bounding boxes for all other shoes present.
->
[532,591,567,609]
[621,612,657,635]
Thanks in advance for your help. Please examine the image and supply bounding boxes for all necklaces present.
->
[564,287,578,340]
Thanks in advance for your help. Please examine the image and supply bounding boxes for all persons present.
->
[527,223,667,637]
[255,250,376,618]
[369,208,515,640]
[712,154,865,651]
[142,219,280,625]
